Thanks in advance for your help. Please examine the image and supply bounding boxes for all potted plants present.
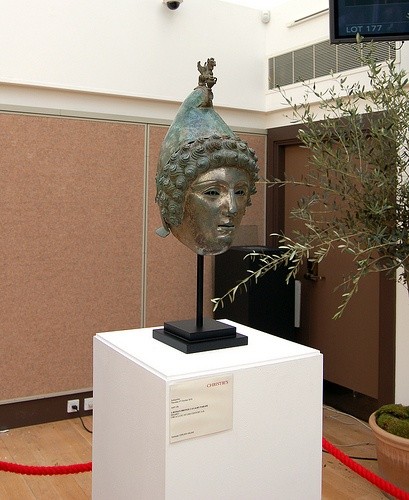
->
[207,31,409,500]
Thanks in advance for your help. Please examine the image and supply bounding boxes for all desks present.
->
[90,317,327,500]
[197,242,297,344]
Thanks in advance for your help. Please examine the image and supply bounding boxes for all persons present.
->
[155,106,259,254]
